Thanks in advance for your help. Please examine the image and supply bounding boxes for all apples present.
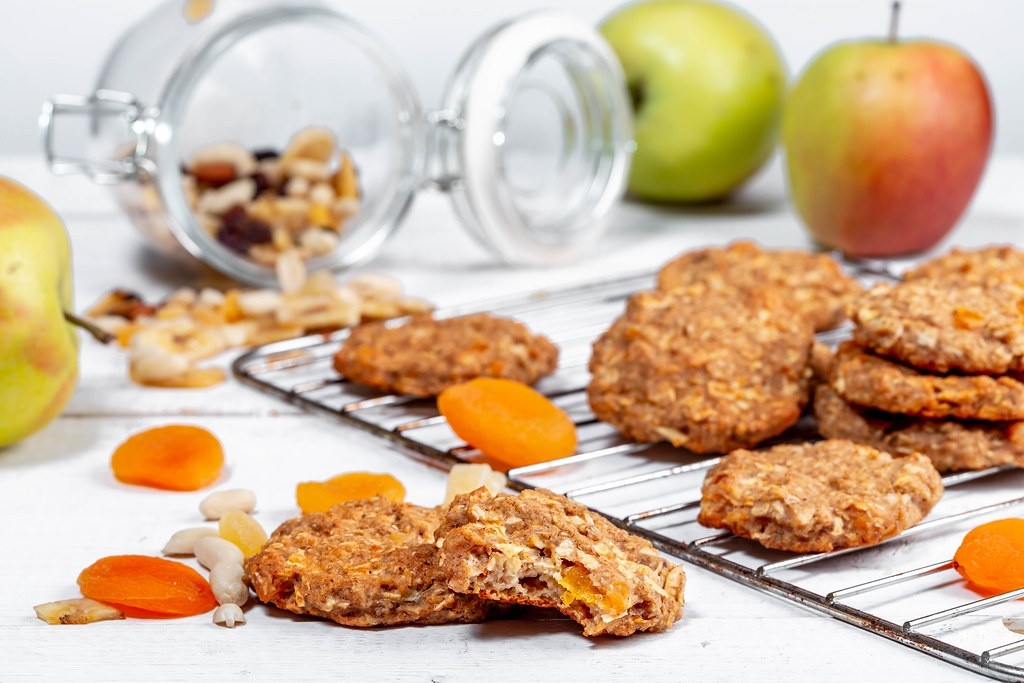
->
[595,0,791,202]
[0,172,115,448]
[785,0,994,259]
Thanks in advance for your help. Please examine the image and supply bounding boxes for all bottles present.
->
[36,0,632,288]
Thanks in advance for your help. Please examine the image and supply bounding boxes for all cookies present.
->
[243,241,1024,639]
